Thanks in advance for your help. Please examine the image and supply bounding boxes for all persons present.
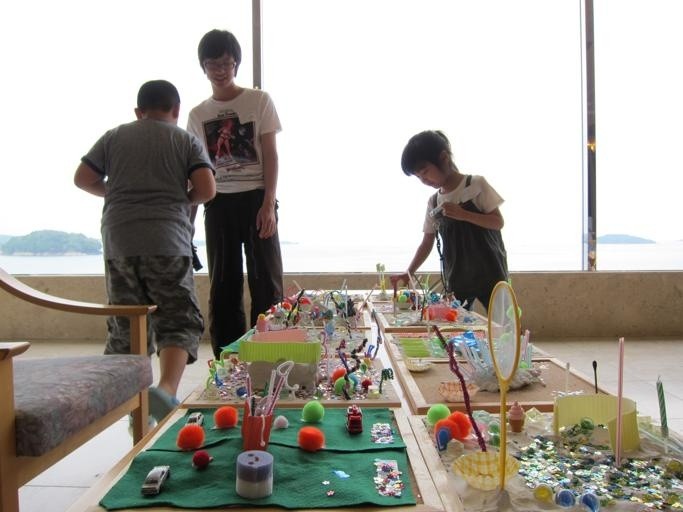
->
[189,28,281,361]
[388,131,512,320]
[74,80,215,433]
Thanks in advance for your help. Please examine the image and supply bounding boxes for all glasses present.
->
[205,63,237,70]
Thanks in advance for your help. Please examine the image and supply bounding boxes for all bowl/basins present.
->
[434,381,478,403]
[453,450,518,490]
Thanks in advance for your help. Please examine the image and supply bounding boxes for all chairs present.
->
[0,267,158,511]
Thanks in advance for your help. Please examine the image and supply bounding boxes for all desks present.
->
[67,289,680,511]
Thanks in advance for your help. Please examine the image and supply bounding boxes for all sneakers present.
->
[128,388,181,437]
